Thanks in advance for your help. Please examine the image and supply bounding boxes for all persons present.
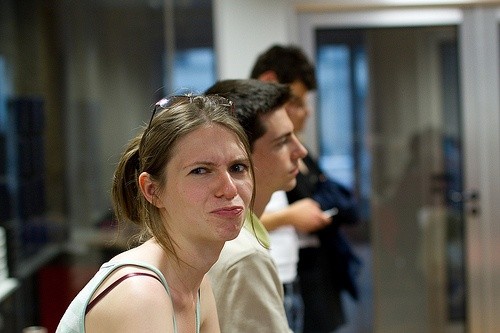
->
[250,44,333,333]
[53,93,272,333]
[202,78,309,333]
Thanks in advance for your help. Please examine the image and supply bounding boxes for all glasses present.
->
[145,94,235,134]
